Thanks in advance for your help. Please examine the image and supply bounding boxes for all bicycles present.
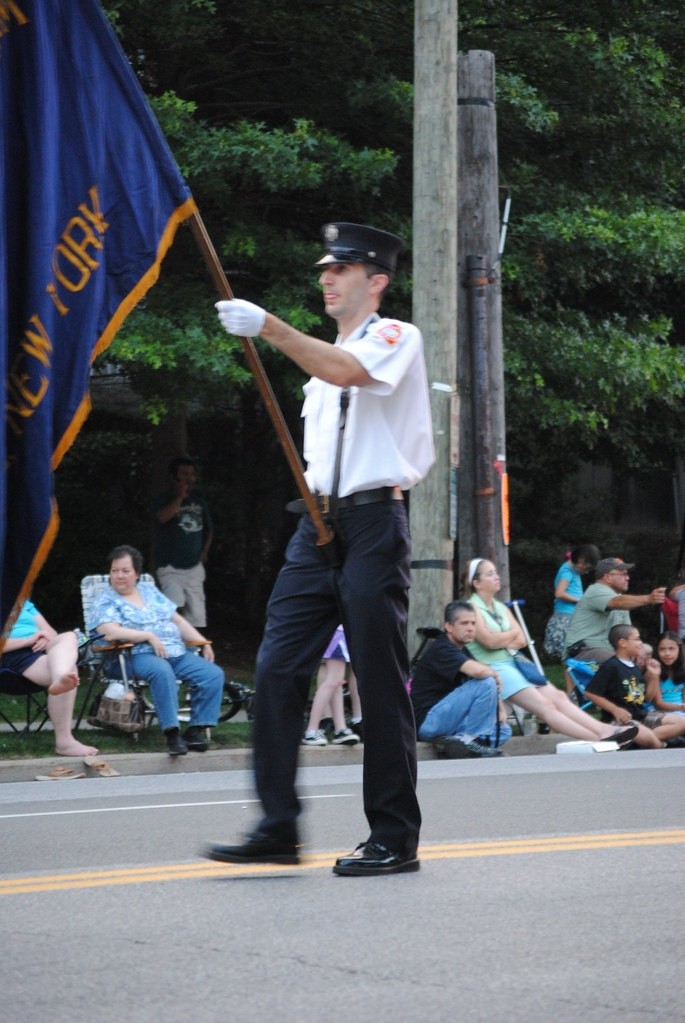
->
[217,677,351,734]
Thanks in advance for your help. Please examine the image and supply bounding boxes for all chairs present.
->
[81,574,217,748]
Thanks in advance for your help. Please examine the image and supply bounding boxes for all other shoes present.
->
[184,733,208,752]
[167,735,186,755]
[433,736,469,759]
[664,737,685,747]
[468,741,502,757]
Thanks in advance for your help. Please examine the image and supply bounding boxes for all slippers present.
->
[34,767,85,781]
[617,739,632,751]
[600,726,637,741]
[83,755,120,776]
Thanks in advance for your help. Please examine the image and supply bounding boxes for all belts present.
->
[287,487,403,514]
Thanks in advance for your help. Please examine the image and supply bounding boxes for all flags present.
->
[0,0,196,639]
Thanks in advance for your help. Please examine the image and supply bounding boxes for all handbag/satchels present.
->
[514,652,547,685]
[88,655,145,733]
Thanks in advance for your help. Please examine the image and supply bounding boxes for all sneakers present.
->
[301,729,328,745]
[331,728,359,745]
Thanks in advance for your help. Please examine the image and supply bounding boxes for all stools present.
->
[566,660,600,709]
[0,627,106,741]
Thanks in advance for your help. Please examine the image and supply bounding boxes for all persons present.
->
[646,631,685,746]
[303,625,362,745]
[199,223,436,878]
[413,600,512,757]
[544,544,600,705]
[669,584,685,643]
[564,558,665,662]
[585,624,685,748]
[152,455,215,637]
[1,598,100,757]
[90,545,225,755]
[461,558,638,748]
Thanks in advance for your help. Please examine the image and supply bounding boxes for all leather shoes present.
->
[208,833,299,864]
[332,840,420,873]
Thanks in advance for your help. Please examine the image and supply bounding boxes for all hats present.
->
[315,221,405,272]
[594,558,635,579]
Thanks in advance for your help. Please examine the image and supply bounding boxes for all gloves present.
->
[214,299,266,338]
